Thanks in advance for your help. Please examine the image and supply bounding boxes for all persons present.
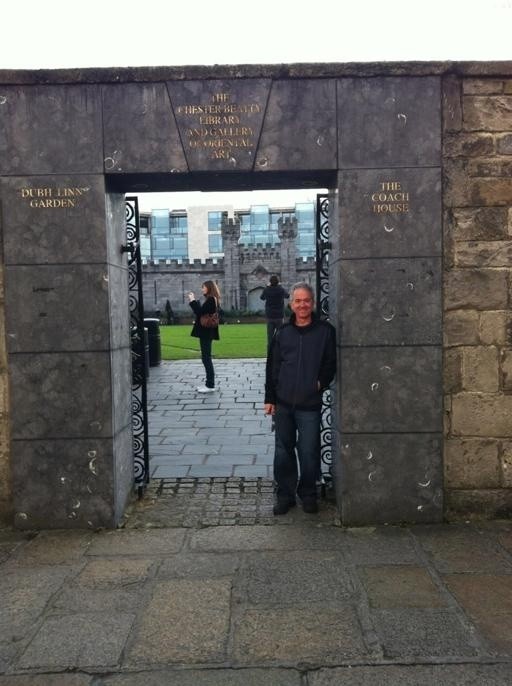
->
[264,282,335,515]
[260,276,290,357]
[187,280,221,393]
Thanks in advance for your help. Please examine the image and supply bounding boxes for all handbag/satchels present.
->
[200,296,220,328]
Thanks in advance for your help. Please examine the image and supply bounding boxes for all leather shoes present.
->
[297,489,317,514]
[274,498,296,514]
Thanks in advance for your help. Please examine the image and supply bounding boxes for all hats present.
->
[269,274,279,281]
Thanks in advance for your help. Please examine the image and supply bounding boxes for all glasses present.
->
[264,412,276,432]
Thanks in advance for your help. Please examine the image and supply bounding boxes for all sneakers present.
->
[197,385,215,392]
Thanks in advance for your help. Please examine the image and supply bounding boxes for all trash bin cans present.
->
[131,318,161,385]
